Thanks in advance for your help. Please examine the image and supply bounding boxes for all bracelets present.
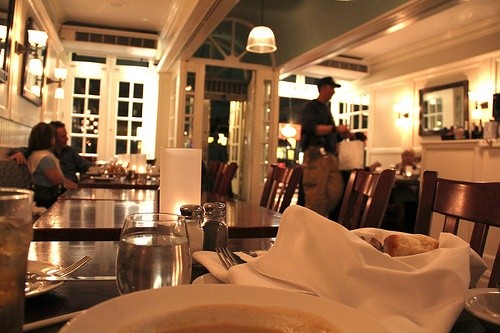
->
[331,125,336,132]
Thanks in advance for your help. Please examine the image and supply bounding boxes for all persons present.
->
[297,76,355,217]
[6,121,105,183]
[208,132,222,160]
[26,122,79,208]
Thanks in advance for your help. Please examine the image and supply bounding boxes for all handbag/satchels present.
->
[337,135,365,170]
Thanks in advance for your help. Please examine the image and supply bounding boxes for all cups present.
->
[0,186,37,333]
[115,213,192,295]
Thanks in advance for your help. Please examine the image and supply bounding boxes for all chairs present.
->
[205,160,239,195]
[260,164,302,214]
[414,171,500,288]
[336,168,397,231]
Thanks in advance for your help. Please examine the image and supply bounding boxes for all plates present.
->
[23,258,65,298]
[462,287,500,326]
[90,176,115,180]
[56,282,387,333]
[190,270,227,285]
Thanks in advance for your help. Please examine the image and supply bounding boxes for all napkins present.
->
[191,205,487,333]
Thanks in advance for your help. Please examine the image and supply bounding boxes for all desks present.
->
[25,176,282,333]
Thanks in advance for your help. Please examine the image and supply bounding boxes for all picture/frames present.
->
[21,17,48,106]
[0,0,16,83]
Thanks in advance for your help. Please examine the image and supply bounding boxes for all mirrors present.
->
[419,80,469,136]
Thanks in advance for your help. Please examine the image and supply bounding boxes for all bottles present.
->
[202,202,228,253]
[463,119,484,140]
[177,204,205,268]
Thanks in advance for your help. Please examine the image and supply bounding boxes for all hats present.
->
[317,77,341,88]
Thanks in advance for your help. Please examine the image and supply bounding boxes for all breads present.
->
[360,233,440,257]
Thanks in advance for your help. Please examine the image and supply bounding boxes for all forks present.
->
[47,255,94,278]
[215,243,240,272]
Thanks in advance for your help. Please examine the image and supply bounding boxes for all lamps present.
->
[47,68,68,99]
[246,0,277,54]
[15,30,48,76]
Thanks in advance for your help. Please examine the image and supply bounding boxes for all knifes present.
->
[24,271,117,282]
